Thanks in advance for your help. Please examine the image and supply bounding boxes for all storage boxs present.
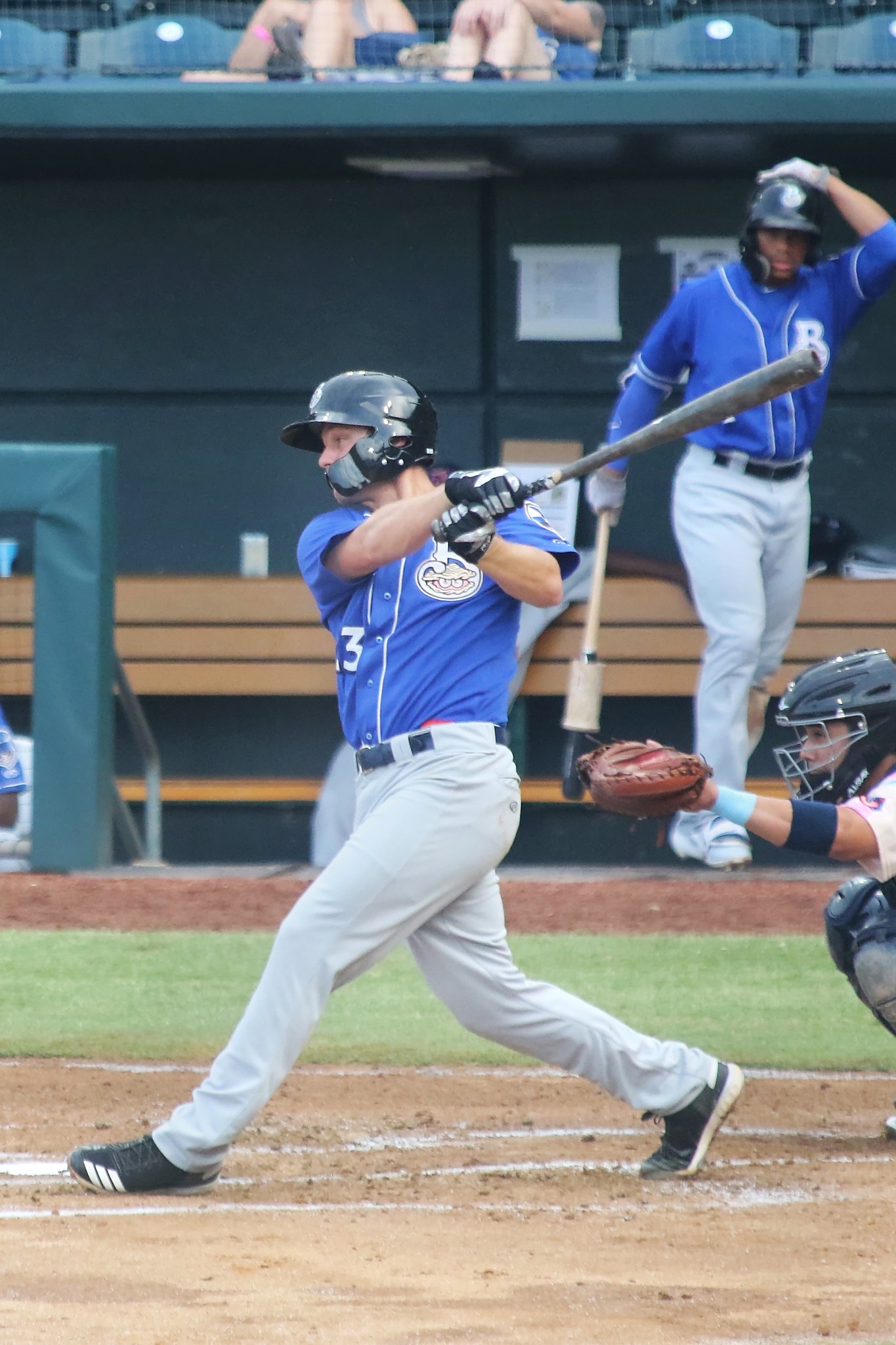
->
[498,436,579,544]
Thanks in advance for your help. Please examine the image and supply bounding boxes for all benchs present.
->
[0,575,896,802]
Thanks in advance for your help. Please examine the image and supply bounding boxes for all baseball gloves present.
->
[575,740,714,818]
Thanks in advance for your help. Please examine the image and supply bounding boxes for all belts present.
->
[356,731,434,773]
[715,453,805,480]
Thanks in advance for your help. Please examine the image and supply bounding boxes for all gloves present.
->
[440,503,492,564]
[582,466,627,530]
[446,468,525,522]
[758,160,830,196]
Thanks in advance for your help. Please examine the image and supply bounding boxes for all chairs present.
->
[1,1,896,78]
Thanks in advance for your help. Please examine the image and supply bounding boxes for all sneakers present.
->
[65,1139,219,1195]
[641,1061,746,1178]
[671,814,753,871]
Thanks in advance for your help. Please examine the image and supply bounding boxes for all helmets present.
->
[737,181,823,286]
[772,649,896,804]
[278,369,438,496]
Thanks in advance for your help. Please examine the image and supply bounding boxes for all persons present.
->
[66,368,747,1195]
[602,644,896,1136]
[586,159,896,869]
[232,0,605,83]
[0,713,29,832]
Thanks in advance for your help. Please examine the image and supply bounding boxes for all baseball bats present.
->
[430,347,825,544]
